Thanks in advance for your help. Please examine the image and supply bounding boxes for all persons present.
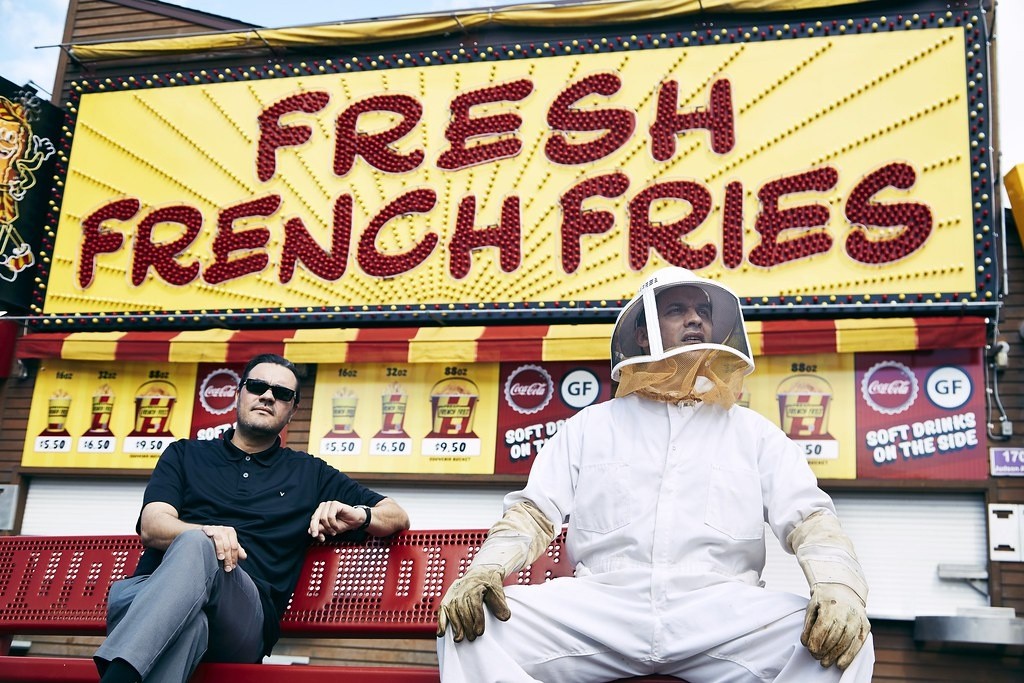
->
[436,267,877,683]
[91,352,412,683]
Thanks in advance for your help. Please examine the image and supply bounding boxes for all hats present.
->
[612,266,755,410]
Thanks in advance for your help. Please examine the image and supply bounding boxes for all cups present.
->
[382,394,407,434]
[90,394,115,432]
[47,398,71,432]
[332,396,357,434]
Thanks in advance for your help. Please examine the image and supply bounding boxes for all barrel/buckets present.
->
[134,379,178,433]
[430,377,479,434]
[775,374,834,436]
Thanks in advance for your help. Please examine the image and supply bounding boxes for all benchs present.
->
[0,526,575,683]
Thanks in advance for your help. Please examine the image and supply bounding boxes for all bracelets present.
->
[353,504,372,532]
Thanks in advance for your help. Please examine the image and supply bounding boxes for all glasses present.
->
[238,378,299,404]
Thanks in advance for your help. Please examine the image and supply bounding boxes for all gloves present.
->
[786,510,872,670]
[437,502,555,643]
